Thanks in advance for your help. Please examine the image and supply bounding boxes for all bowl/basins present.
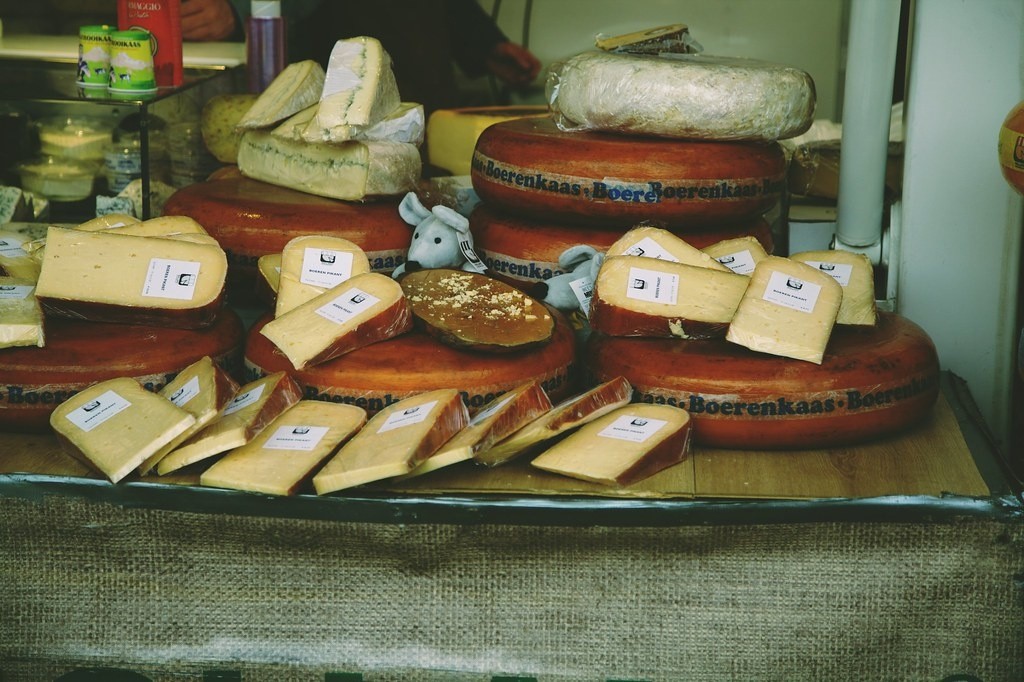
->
[10,114,217,204]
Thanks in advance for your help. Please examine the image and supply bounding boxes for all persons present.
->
[181,0,323,41]
[286,0,542,113]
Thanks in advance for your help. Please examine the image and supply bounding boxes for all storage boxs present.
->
[0,63,247,221]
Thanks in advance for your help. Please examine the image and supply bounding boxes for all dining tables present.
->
[0,370,1024,682]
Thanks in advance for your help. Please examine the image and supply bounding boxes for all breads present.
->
[0,24,941,488]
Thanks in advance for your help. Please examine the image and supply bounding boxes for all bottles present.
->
[117,0,185,89]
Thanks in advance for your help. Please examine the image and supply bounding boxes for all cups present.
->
[106,30,159,92]
[75,25,117,86]
[243,16,286,94]
